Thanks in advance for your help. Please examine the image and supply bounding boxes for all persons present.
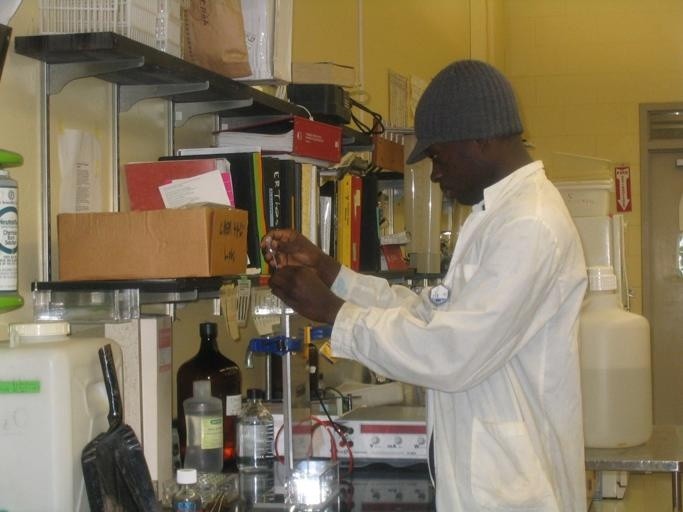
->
[259,58,589,512]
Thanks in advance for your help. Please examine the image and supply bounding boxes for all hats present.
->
[405,59,524,165]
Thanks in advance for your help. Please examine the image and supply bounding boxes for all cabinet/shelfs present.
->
[6,28,435,292]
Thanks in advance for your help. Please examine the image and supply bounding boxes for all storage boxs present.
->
[56,201,250,287]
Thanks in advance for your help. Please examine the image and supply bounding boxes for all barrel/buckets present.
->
[574,267,652,447]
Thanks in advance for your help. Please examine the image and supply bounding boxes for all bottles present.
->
[232,388,275,475]
[170,467,205,512]
[179,378,225,475]
[0,169,18,296]
[174,321,240,472]
